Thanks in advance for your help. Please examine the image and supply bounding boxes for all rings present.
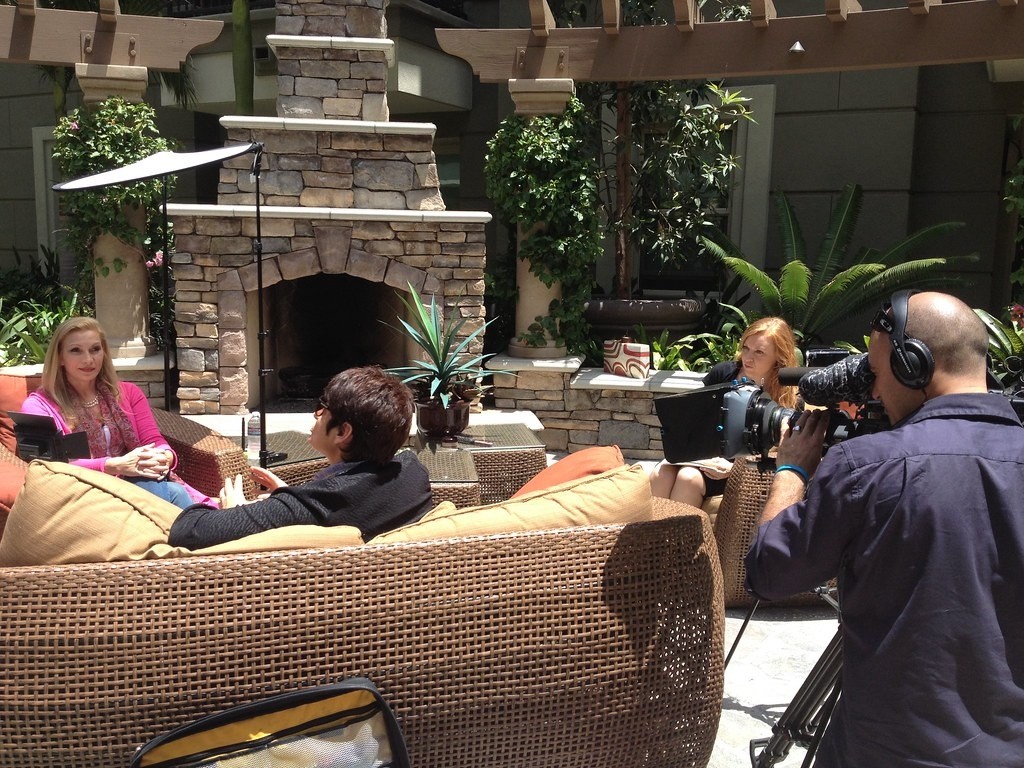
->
[161,471,163,475]
[792,426,801,431]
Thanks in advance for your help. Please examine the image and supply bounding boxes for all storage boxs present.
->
[604,340,650,379]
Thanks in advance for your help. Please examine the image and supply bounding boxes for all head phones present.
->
[870,289,935,390]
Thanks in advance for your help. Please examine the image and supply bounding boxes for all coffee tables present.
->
[233,425,546,510]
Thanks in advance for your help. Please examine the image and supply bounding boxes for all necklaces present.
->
[84,394,99,407]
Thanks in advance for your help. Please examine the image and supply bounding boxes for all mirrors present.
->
[0,462,28,509]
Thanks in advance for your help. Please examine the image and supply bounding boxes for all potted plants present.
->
[547,0,761,364]
[376,280,521,436]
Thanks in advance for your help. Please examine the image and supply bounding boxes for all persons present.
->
[19,317,219,510]
[166,368,433,551]
[648,318,798,510]
[742,291,1024,768]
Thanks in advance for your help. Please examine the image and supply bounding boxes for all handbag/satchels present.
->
[129,678,411,768]
[604,337,650,378]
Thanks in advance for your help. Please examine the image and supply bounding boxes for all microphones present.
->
[778,366,827,386]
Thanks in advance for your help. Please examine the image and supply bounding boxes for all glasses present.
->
[315,395,332,416]
[869,303,914,342]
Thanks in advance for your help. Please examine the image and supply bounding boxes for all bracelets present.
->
[774,464,809,485]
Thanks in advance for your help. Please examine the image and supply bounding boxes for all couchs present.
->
[701,451,838,610]
[0,408,256,505]
[0,458,728,767]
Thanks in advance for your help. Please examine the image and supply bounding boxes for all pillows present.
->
[508,444,625,499]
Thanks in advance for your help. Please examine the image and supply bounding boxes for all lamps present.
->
[789,41,805,53]
